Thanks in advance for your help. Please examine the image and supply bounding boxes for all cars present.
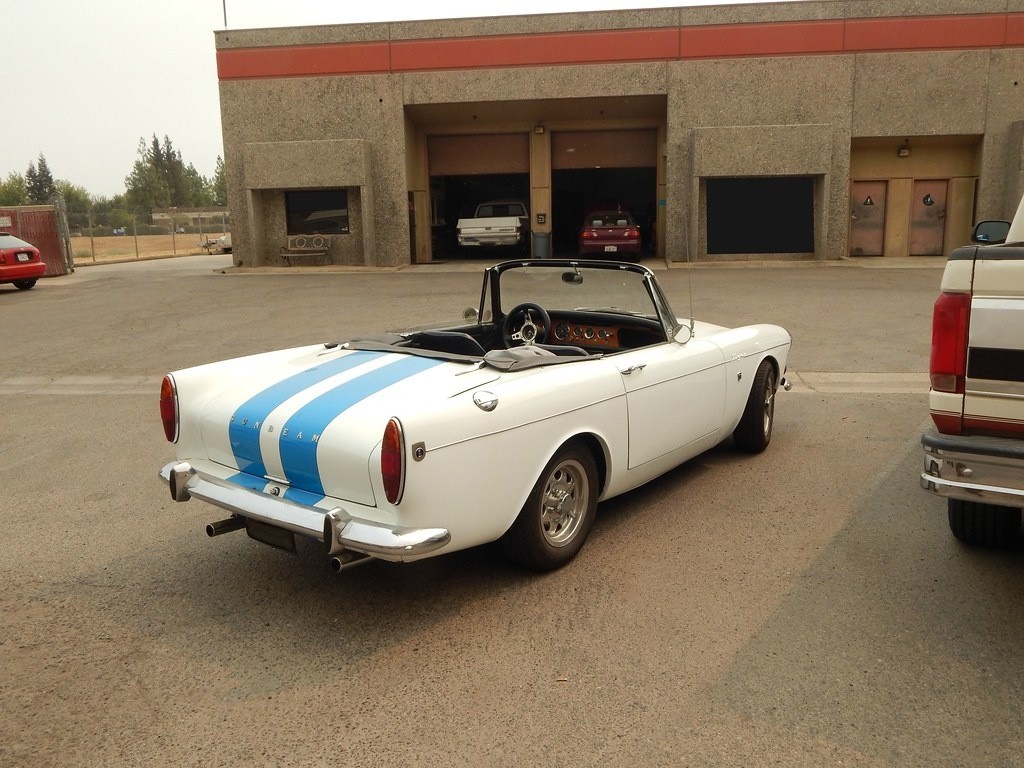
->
[0,232,47,291]
[577,211,643,262]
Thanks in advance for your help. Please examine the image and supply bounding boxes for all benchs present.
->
[280,235,334,266]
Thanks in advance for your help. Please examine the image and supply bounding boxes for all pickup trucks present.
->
[455,199,531,258]
[918,195,1023,549]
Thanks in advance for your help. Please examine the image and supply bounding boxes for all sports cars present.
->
[157,258,795,574]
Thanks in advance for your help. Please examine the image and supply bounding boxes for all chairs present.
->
[533,343,589,356]
[418,331,487,356]
[593,219,602,226]
[617,219,627,226]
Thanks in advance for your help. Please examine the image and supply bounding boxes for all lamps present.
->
[898,146,912,157]
[534,126,545,134]
